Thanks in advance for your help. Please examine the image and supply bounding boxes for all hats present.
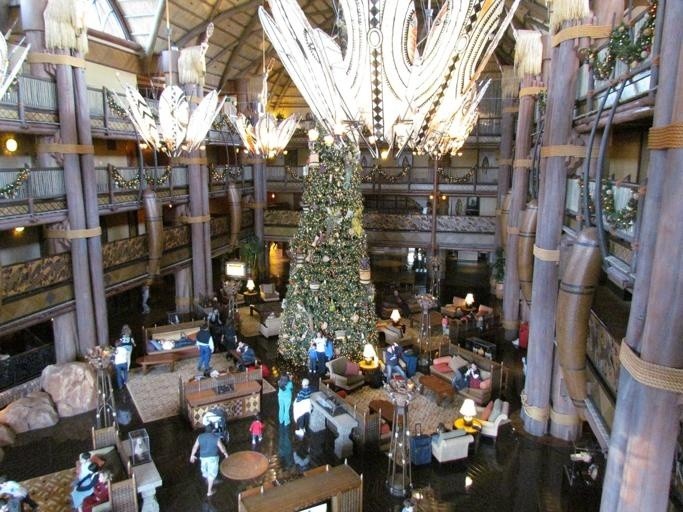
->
[301,378,310,385]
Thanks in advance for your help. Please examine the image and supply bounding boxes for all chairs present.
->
[0,284,513,511]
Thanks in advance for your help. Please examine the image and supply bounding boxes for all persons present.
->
[248,415,264,444]
[141,284,151,315]
[463,362,482,390]
[394,294,411,319]
[276,421,291,471]
[70,462,99,510]
[577,446,599,486]
[119,324,136,373]
[70,452,91,489]
[291,435,313,476]
[188,423,227,496]
[276,374,293,426]
[0,498,21,511]
[383,341,424,395]
[195,323,214,371]
[422,202,431,214]
[0,475,40,510]
[292,377,313,436]
[110,339,130,391]
[401,498,417,511]
[207,307,221,338]
[219,316,237,360]
[80,467,113,512]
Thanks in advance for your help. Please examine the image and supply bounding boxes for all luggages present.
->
[412,423,432,465]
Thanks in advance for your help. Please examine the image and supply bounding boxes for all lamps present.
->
[221,24,305,160]
[107,1,226,159]
[256,0,527,162]
